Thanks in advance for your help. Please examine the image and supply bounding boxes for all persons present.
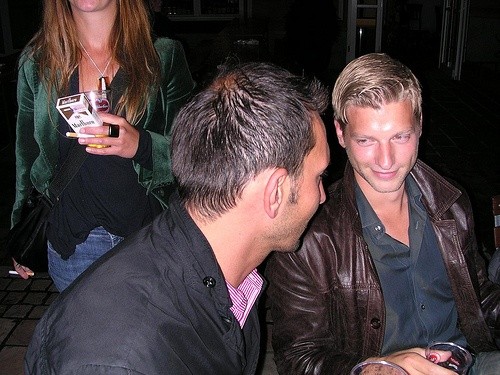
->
[267,52,500,375]
[286,0,338,74]
[7,0,197,294]
[25,61,330,375]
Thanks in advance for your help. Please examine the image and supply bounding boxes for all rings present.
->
[107,124,120,138]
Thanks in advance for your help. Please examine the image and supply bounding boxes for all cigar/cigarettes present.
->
[9,271,34,276]
[66,132,95,139]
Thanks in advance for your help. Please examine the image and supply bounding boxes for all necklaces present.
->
[78,40,113,80]
